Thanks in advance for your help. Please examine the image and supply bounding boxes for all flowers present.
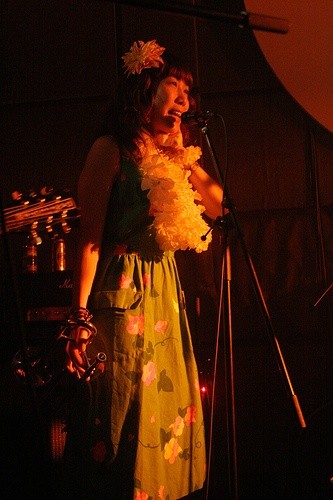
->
[119,38,166,78]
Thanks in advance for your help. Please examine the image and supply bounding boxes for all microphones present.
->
[181,111,218,123]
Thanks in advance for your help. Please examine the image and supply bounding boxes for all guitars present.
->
[0,185,79,246]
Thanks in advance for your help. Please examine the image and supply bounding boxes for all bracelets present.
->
[171,144,203,173]
[59,306,97,343]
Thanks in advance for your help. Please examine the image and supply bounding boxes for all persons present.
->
[57,40,229,500]
